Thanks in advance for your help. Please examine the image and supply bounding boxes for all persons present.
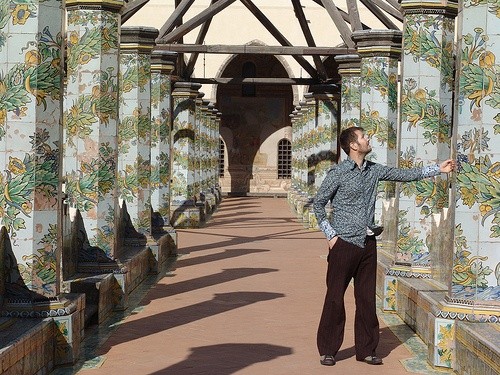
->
[313,126,457,366]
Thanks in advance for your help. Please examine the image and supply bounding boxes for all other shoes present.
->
[320,355,336,365]
[356,355,382,365]
